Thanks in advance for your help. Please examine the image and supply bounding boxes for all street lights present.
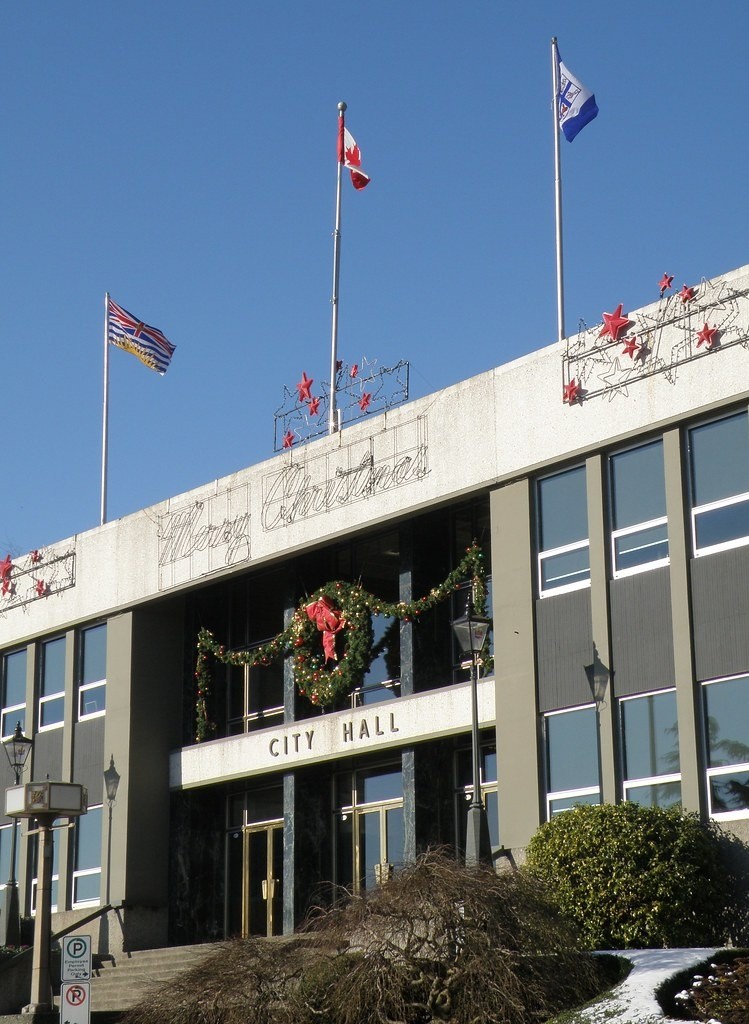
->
[452,591,498,916]
[584,642,614,811]
[102,753,121,906]
[0,721,36,950]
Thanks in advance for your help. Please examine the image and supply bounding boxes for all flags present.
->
[556,47,599,143]
[108,300,177,376]
[338,117,371,190]
